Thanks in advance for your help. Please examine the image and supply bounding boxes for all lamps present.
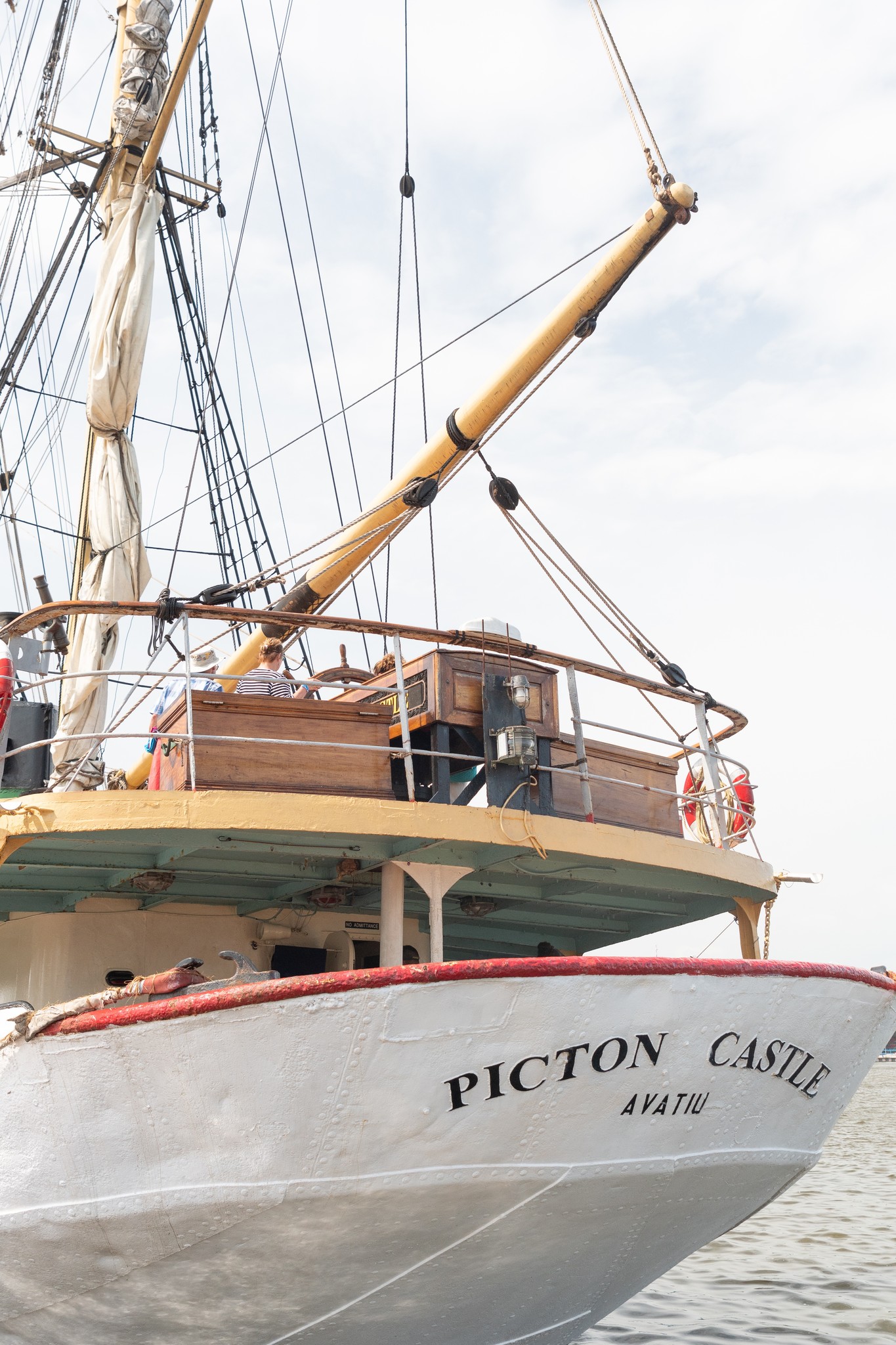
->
[487,722,540,771]
[502,674,533,709]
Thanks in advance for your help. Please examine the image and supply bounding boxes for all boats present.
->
[6,0,895,1342]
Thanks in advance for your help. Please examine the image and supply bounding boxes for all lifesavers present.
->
[681,754,756,851]
